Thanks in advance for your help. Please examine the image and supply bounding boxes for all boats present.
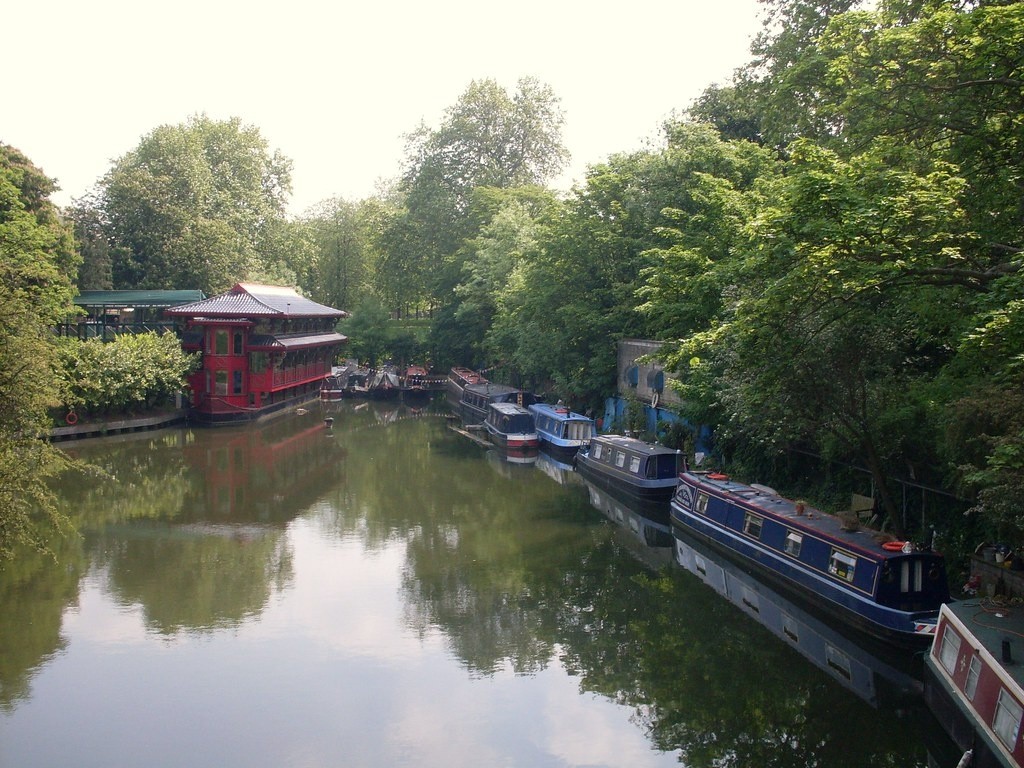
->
[667,518,925,715]
[570,454,677,550]
[528,400,598,455]
[474,422,538,465]
[572,434,688,495]
[668,455,952,654]
[534,434,576,486]
[319,360,540,451]
[922,596,1024,768]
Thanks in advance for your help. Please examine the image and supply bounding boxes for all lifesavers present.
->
[707,474,727,479]
[882,542,905,550]
[651,392,659,408]
[66,413,78,425]
